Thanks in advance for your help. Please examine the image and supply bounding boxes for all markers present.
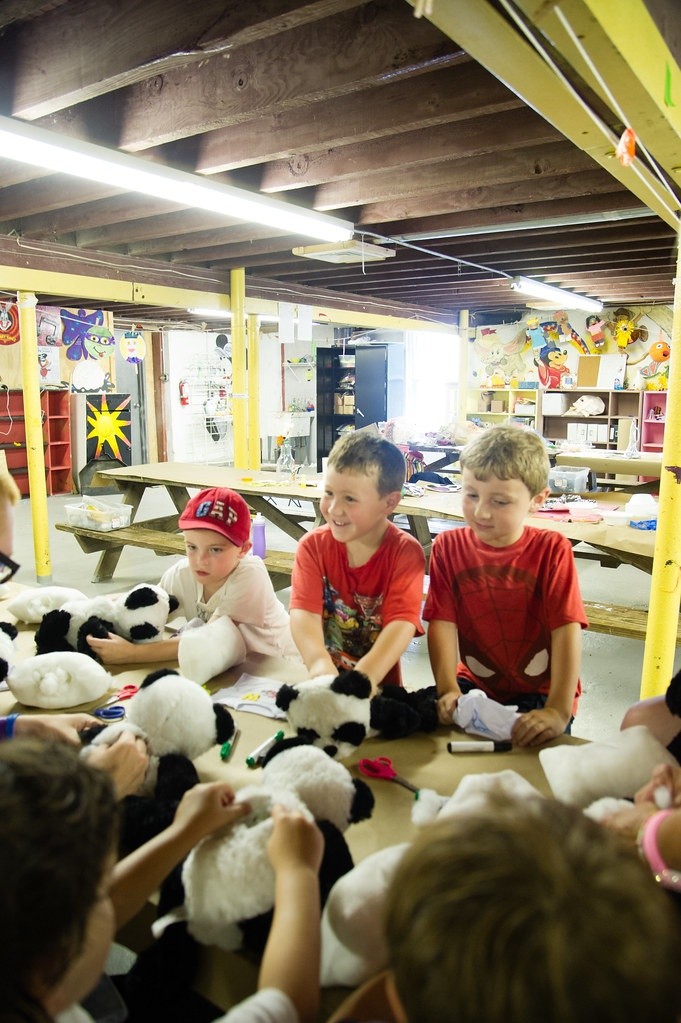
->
[447,741,513,754]
[246,731,286,767]
[220,730,238,759]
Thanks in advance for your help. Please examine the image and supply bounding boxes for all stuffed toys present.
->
[275,670,447,761]
[76,666,236,876]
[153,738,376,958]
[34,582,184,658]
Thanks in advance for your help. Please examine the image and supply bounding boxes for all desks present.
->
[0,579,594,1013]
[395,442,662,491]
[92,460,658,583]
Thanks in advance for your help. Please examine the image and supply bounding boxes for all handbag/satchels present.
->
[403,450,423,482]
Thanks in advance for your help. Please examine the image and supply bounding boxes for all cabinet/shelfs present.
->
[541,389,644,479]
[638,391,667,496]
[314,342,406,473]
[0,388,74,500]
[465,388,544,497]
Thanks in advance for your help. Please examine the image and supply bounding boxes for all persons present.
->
[377,422,587,746]
[0,710,151,803]
[608,653,680,888]
[382,798,681,1023]
[290,430,425,699]
[0,737,325,1023]
[0,454,21,583]
[86,488,308,671]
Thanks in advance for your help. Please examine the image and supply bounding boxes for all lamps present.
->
[0,115,355,243]
[507,276,604,313]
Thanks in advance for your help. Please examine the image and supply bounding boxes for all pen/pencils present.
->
[298,483,317,487]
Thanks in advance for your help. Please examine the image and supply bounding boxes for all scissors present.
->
[358,756,420,793]
[64,705,125,720]
[90,684,138,710]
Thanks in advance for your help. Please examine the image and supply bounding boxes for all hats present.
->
[177,487,251,547]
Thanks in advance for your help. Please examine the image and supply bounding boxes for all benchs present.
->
[586,477,649,488]
[56,522,649,640]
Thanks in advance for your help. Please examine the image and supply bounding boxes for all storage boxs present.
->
[547,465,591,493]
[541,393,570,415]
[566,423,609,442]
[338,354,355,368]
[491,400,505,413]
[477,392,494,412]
[64,501,134,533]
[609,424,618,442]
[514,404,535,415]
[333,393,355,414]
